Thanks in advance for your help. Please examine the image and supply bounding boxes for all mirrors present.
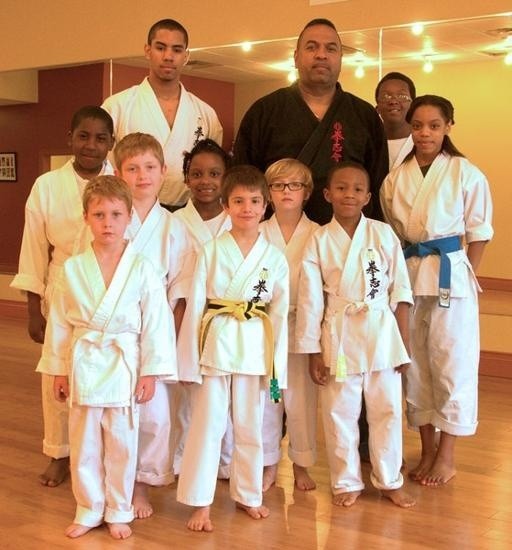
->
[377,10,512,319]
[0,56,112,276]
[109,24,382,162]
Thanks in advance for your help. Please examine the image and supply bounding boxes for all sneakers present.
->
[360,445,405,468]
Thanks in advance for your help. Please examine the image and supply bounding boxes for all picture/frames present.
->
[1,152,18,182]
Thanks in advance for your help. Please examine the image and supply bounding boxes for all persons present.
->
[375,72,416,172]
[233,18,389,227]
[114,133,182,517]
[100,19,222,212]
[35,174,176,539]
[257,158,323,492]
[379,96,494,488]
[175,164,288,532]
[293,159,418,509]
[9,105,117,488]
[170,139,234,479]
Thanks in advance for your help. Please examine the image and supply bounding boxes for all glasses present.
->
[377,95,412,103]
[269,182,307,192]
